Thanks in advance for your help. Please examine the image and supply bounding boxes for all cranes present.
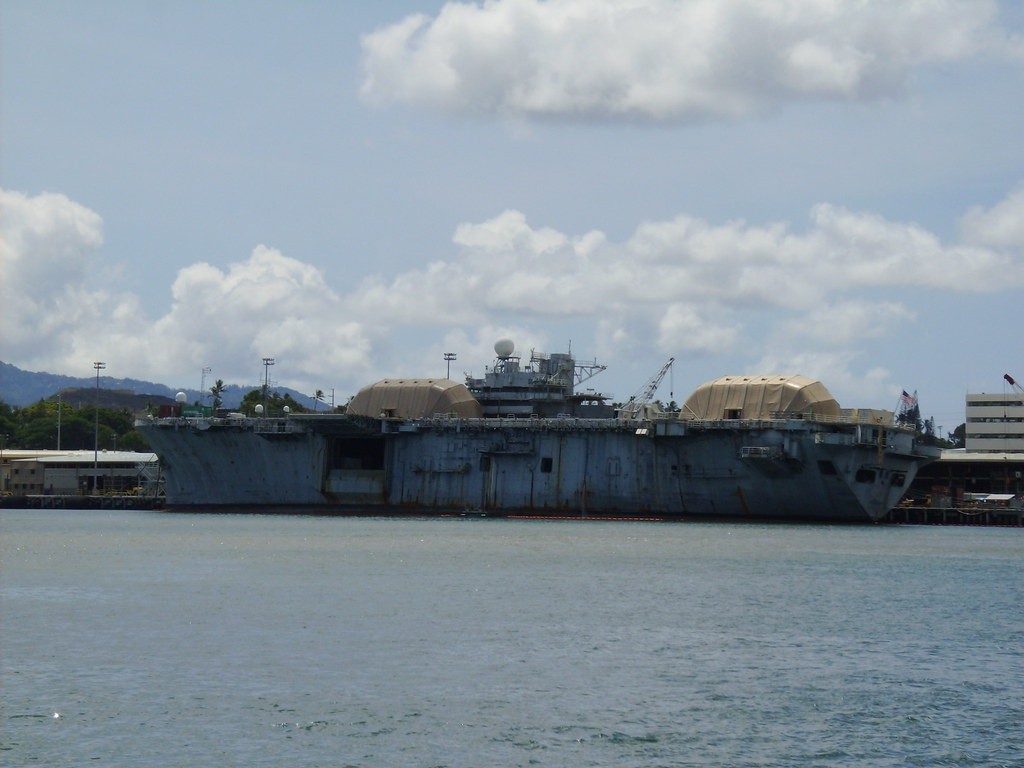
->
[618,357,676,418]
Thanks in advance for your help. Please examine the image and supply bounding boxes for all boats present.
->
[134,334,947,527]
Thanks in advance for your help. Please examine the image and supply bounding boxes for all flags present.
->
[902,391,915,404]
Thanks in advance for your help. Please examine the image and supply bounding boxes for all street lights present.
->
[260,358,277,418]
[90,361,107,495]
[443,352,458,382]
